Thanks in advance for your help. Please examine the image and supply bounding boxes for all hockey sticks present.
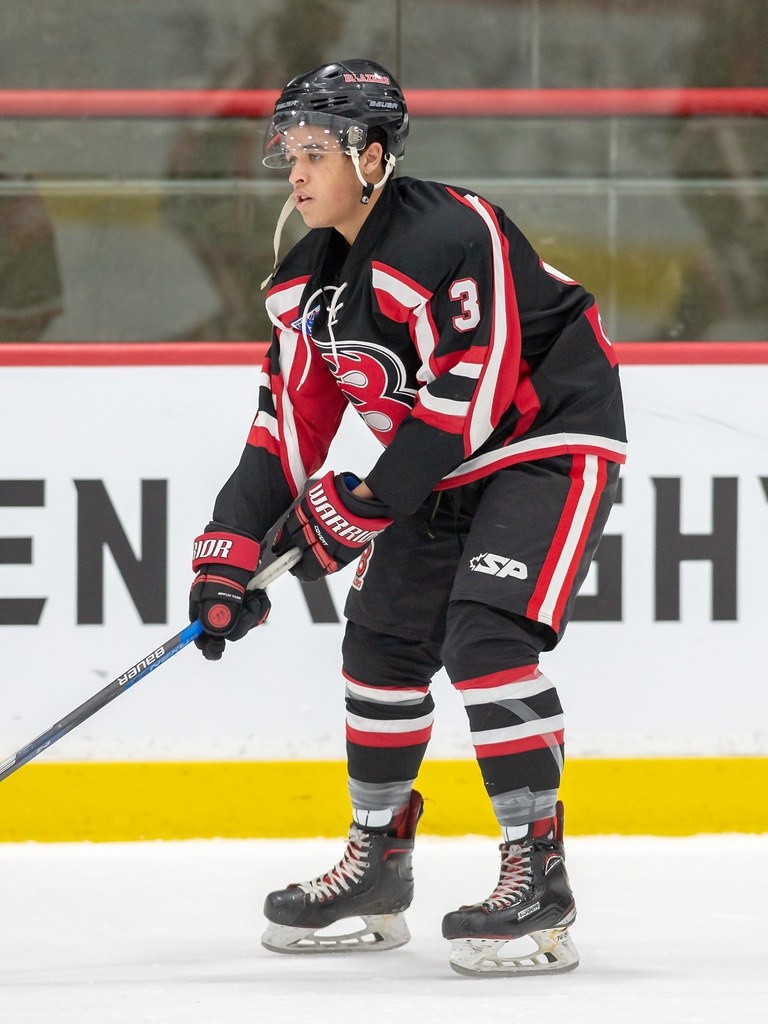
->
[0,546,299,783]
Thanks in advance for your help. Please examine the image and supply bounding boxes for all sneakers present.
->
[261,789,424,954]
[442,801,579,977]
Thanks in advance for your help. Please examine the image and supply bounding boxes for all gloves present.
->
[189,521,271,660]
[271,471,400,583]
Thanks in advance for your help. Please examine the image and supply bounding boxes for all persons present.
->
[186,59,630,976]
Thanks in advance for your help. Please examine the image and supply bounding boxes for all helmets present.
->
[271,59,409,162]
